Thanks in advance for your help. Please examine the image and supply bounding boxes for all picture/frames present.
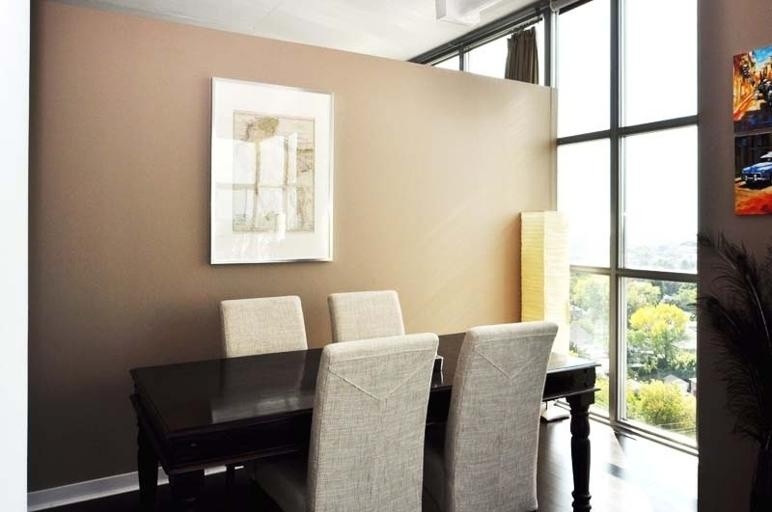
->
[208,77,335,265]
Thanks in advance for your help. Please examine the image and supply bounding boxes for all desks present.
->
[127,330,603,512]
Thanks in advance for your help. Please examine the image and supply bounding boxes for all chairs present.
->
[243,331,440,510]
[326,288,407,345]
[221,294,309,358]
[424,320,558,511]
